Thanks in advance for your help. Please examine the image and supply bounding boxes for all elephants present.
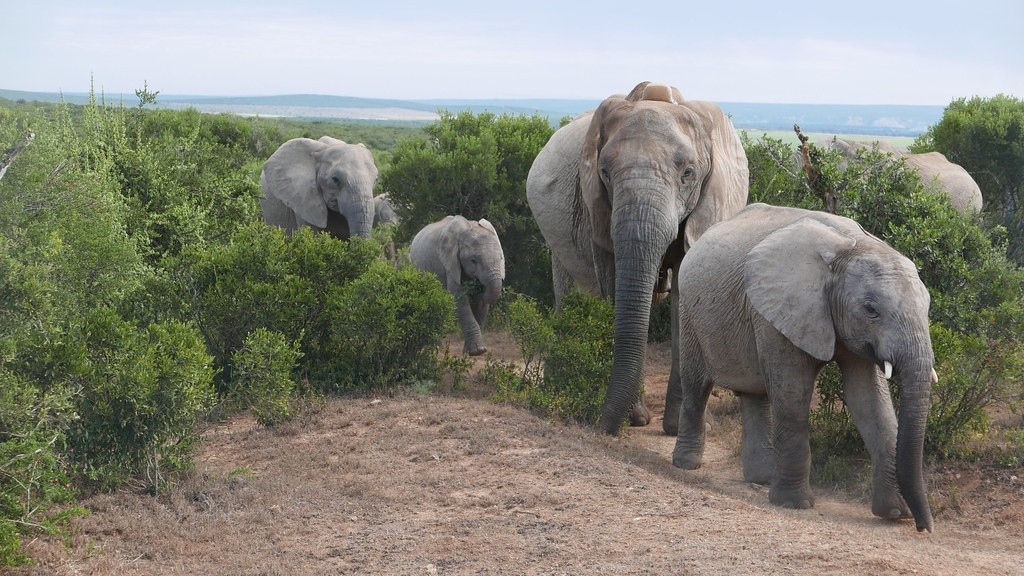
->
[255,82,985,536]
